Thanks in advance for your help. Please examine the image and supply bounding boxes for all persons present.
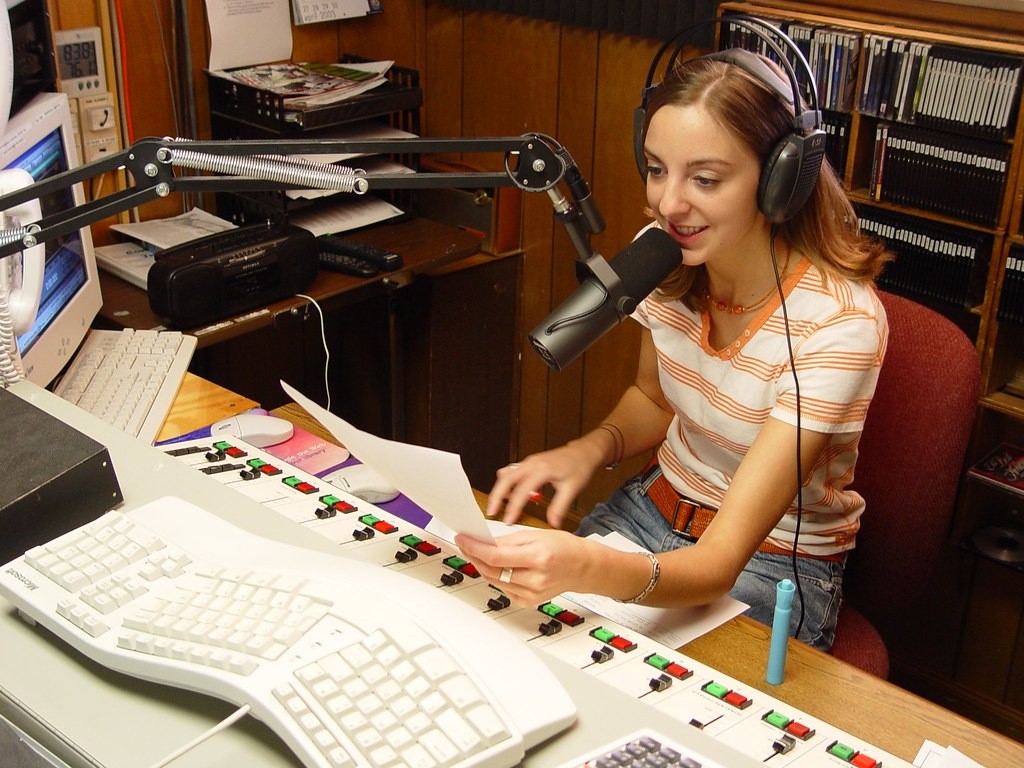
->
[453,44,893,650]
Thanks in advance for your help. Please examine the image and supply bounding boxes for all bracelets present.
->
[599,423,625,470]
[613,551,661,604]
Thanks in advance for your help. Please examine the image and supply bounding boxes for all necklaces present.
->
[702,239,793,315]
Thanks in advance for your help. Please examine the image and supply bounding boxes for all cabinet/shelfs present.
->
[714,0,1024,573]
[207,53,424,237]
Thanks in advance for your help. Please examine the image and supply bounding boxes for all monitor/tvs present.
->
[0,91,104,390]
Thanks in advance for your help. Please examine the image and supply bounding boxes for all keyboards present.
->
[52,327,198,447]
[558,728,730,768]
[0,495,578,768]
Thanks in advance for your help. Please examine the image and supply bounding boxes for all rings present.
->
[509,462,520,470]
[500,567,514,583]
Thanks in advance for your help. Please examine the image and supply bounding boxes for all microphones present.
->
[527,228,684,373]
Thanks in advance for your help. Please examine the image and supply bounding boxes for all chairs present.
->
[824,288,982,681]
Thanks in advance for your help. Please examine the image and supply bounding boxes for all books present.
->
[806,113,1011,230]
[724,7,1024,137]
[994,243,1024,323]
[840,202,988,328]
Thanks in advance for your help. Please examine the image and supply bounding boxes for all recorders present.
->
[147,223,319,330]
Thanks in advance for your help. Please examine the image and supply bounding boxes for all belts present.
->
[641,454,846,562]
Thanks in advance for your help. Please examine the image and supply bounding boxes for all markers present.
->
[765,578,796,685]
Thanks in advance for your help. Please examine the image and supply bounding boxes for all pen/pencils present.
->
[456,222,487,238]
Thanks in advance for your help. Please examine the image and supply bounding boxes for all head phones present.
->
[633,13,828,226]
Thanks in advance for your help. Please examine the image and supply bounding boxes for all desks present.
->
[267,400,1024,768]
[98,217,483,444]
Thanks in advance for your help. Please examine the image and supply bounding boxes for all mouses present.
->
[210,413,294,449]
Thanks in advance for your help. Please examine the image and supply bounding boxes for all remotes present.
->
[325,241,404,272]
[317,252,380,278]
